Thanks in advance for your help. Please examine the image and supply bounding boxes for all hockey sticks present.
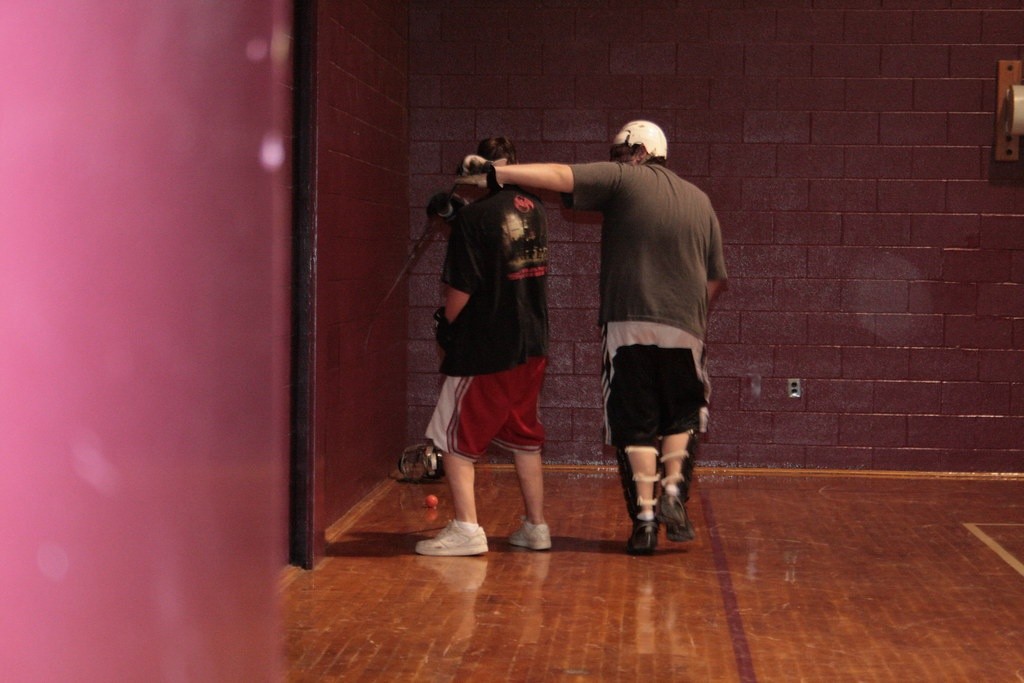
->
[364,158,479,351]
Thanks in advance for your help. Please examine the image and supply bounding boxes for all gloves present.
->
[454,155,508,187]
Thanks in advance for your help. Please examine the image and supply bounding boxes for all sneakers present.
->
[508,515,550,550]
[415,519,489,555]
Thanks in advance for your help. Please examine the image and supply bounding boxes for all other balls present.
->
[426,495,438,508]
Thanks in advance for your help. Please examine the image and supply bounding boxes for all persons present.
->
[453,120,728,554]
[415,136,552,557]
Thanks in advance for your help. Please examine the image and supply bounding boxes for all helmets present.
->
[613,120,668,161]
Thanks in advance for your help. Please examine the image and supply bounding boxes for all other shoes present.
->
[656,496,694,542]
[627,518,655,551]
